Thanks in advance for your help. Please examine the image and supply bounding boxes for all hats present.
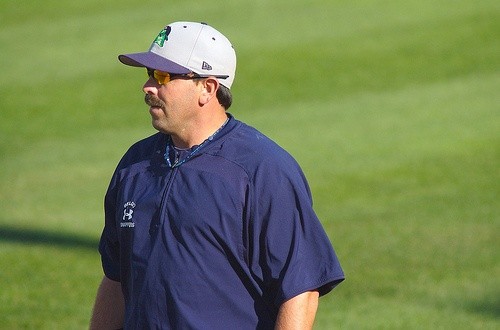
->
[118,22,237,89]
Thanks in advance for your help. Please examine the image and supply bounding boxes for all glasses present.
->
[146,68,228,84]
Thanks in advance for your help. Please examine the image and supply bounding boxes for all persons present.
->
[89,20,345,330]
